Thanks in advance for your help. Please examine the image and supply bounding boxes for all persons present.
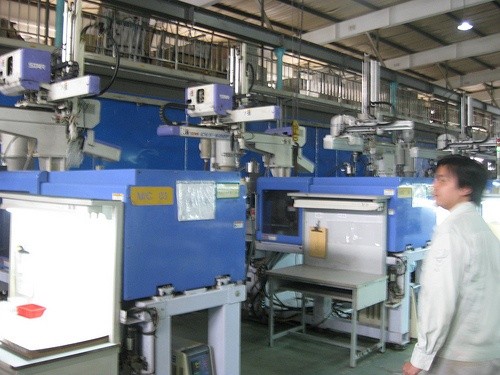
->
[400,154,500,375]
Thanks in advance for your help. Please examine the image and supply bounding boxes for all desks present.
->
[0,299,120,374]
[263,262,390,367]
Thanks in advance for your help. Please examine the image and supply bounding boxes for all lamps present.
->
[456,0,473,31]
